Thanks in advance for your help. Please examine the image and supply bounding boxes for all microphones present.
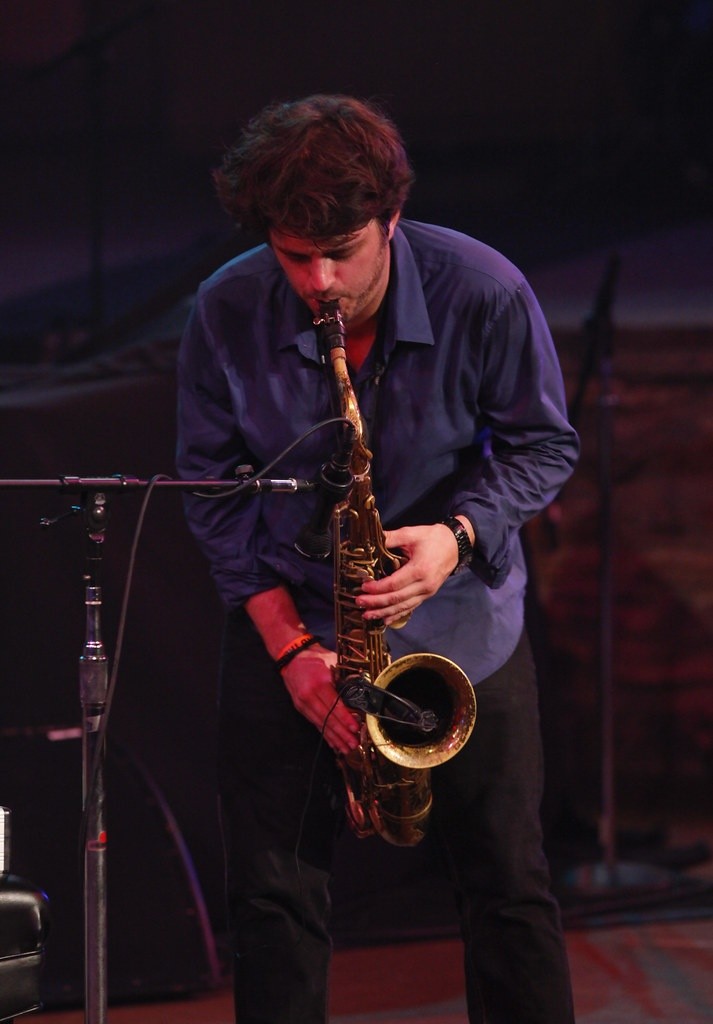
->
[293,441,355,564]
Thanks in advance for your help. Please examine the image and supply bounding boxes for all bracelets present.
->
[273,631,323,673]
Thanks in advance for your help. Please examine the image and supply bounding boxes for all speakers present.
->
[0,716,222,1009]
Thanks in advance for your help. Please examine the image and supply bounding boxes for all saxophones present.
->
[307,296,477,851]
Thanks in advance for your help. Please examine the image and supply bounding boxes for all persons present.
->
[178,94,578,1024]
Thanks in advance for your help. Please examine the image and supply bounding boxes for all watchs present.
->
[439,514,471,577]
